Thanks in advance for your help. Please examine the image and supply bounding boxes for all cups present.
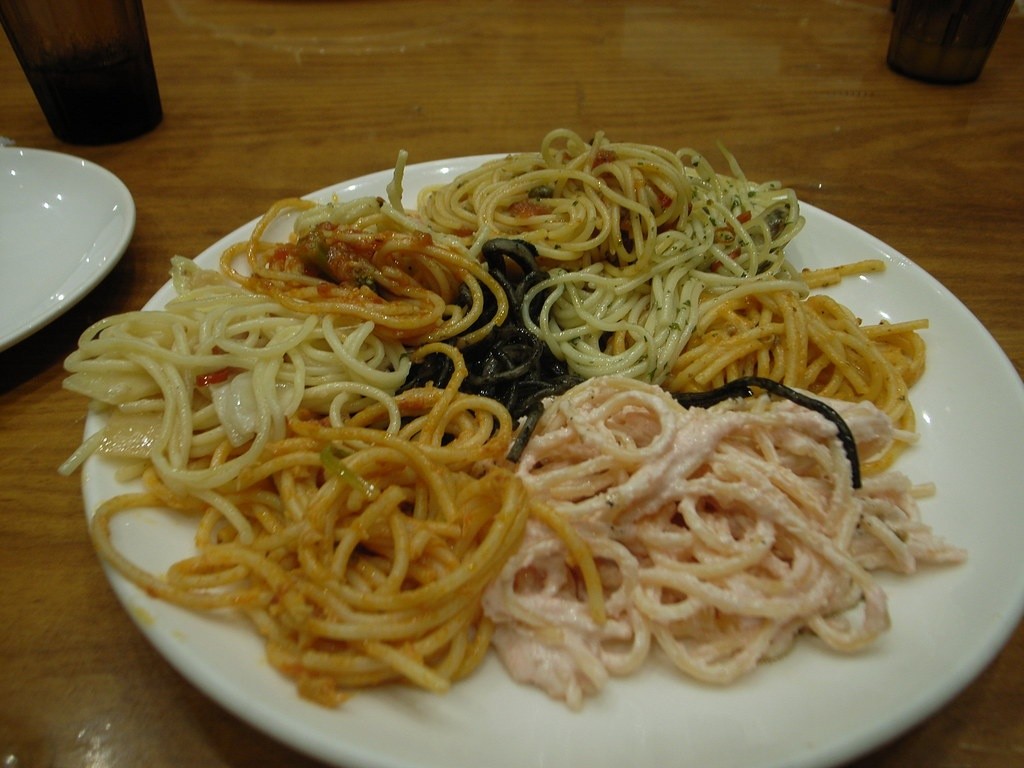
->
[886,0,1015,85]
[0,0,164,146]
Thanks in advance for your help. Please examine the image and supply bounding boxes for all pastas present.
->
[58,128,969,712]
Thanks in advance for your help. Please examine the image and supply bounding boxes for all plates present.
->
[79,152,1024,768]
[0,144,136,352]
